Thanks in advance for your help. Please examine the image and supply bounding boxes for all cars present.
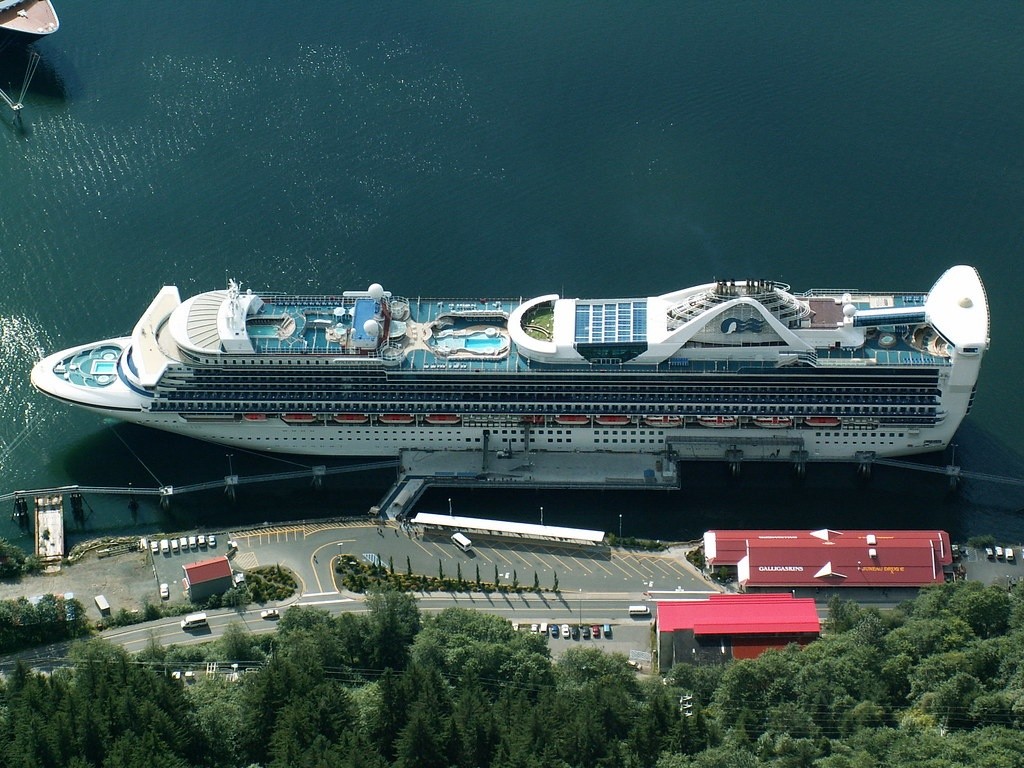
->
[160,583,169,601]
[986,546,1014,561]
[151,535,216,553]
[261,610,278,618]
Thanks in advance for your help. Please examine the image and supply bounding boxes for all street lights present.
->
[619,514,622,537]
[540,507,543,525]
[449,498,452,516]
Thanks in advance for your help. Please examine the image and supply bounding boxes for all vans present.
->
[629,606,651,617]
[451,533,471,552]
[531,623,611,639]
[181,613,207,630]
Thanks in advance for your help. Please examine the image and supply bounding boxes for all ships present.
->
[31,241,989,497]
